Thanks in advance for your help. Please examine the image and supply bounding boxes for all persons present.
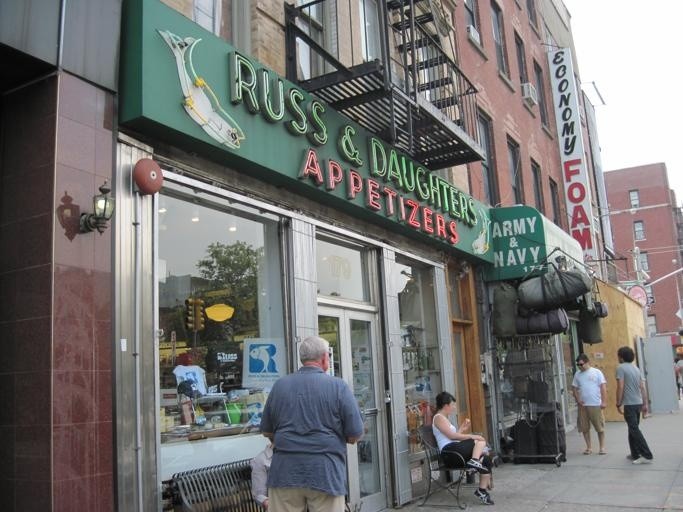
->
[675,358,683,392]
[260,338,365,512]
[673,357,680,399]
[614,346,653,464]
[432,391,495,505]
[571,354,607,456]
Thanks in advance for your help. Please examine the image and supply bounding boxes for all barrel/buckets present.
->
[223,403,241,422]
[513,420,538,463]
[538,411,566,463]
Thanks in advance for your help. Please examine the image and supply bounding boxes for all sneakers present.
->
[627,454,640,461]
[474,487,494,504]
[632,456,655,464]
[466,459,489,472]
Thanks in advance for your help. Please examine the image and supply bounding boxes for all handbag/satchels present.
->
[491,336,567,464]
[491,260,608,346]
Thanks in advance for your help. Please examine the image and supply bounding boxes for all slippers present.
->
[583,448,607,455]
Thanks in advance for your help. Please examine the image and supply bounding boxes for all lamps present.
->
[75,179,116,237]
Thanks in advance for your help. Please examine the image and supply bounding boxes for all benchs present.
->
[171,457,364,512]
[409,424,501,511]
